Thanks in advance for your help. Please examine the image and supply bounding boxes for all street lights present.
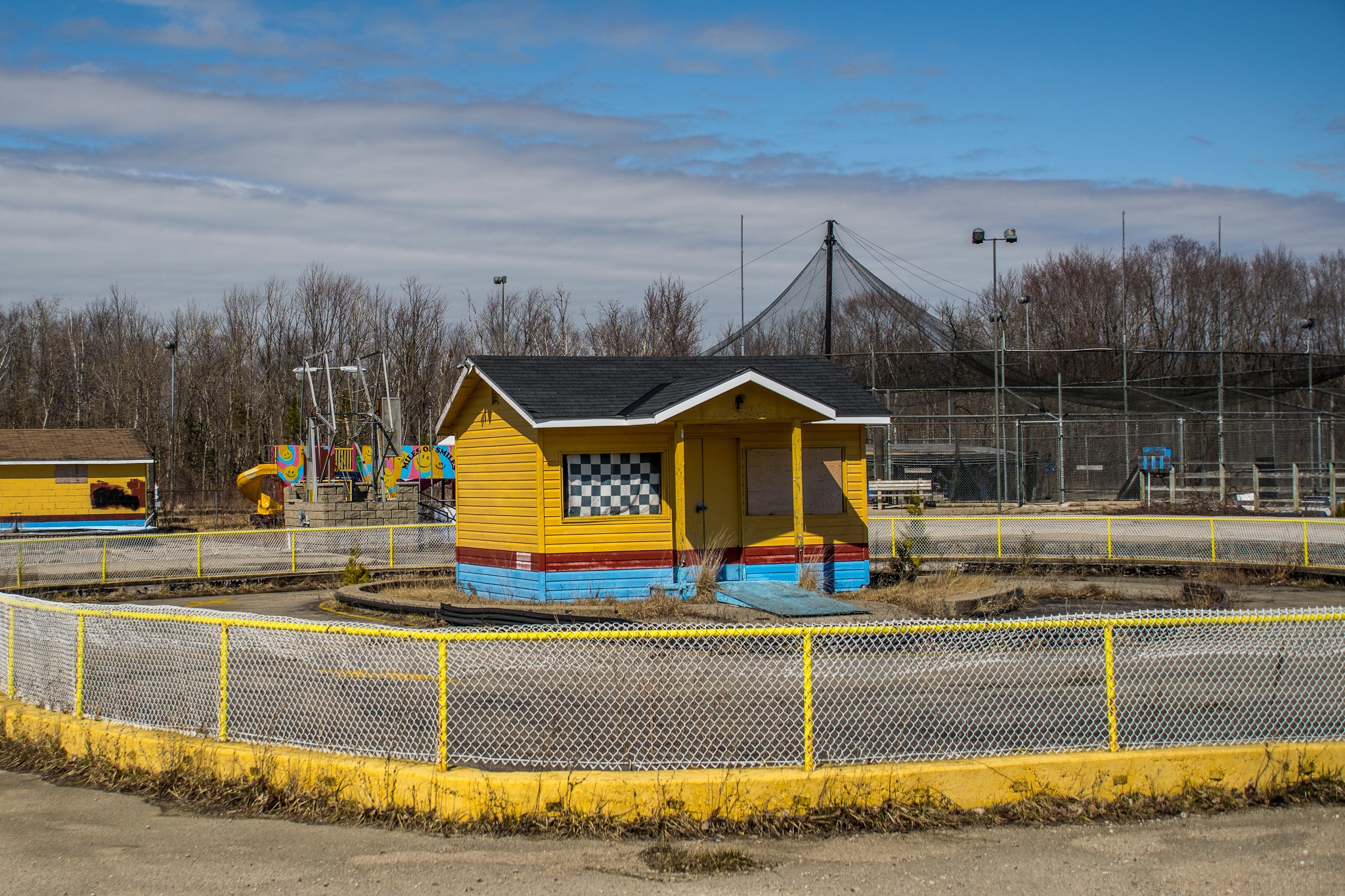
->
[988,310,1008,503]
[1298,317,1316,497]
[1019,293,1032,386]
[972,228,1019,514]
[293,365,368,483]
[164,341,178,525]
[493,275,507,355]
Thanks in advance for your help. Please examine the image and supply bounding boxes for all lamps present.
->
[457,361,469,369]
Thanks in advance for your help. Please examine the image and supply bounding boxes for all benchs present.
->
[868,479,932,509]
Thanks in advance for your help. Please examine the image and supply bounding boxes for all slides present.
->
[237,463,284,516]
